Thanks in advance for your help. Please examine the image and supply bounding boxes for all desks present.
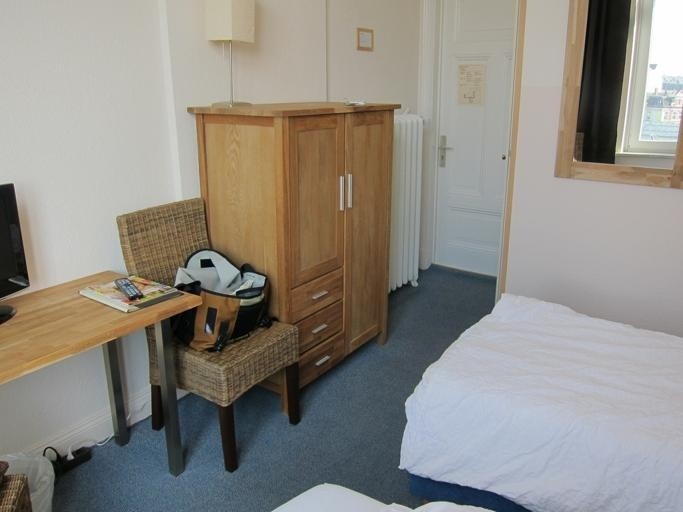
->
[0,271,203,477]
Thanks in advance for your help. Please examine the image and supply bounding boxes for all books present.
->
[77,275,183,313]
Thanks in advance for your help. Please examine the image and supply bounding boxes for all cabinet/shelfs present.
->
[187,101,401,413]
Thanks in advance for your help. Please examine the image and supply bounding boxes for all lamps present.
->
[201,0,255,111]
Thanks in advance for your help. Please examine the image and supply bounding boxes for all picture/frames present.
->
[355,27,374,51]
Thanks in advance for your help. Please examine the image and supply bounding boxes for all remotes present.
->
[115,278,143,300]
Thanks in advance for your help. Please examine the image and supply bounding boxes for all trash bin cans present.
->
[0,455,56,511]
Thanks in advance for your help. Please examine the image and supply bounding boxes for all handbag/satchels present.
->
[170,249,279,352]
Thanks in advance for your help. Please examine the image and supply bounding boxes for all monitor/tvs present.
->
[0,184,30,325]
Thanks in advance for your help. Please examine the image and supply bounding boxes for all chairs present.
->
[116,197,301,473]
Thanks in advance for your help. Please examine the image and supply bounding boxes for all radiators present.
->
[387,113,420,294]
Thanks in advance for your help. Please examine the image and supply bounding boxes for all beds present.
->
[399,294,682,512]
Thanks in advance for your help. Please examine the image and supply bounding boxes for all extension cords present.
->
[50,446,92,476]
